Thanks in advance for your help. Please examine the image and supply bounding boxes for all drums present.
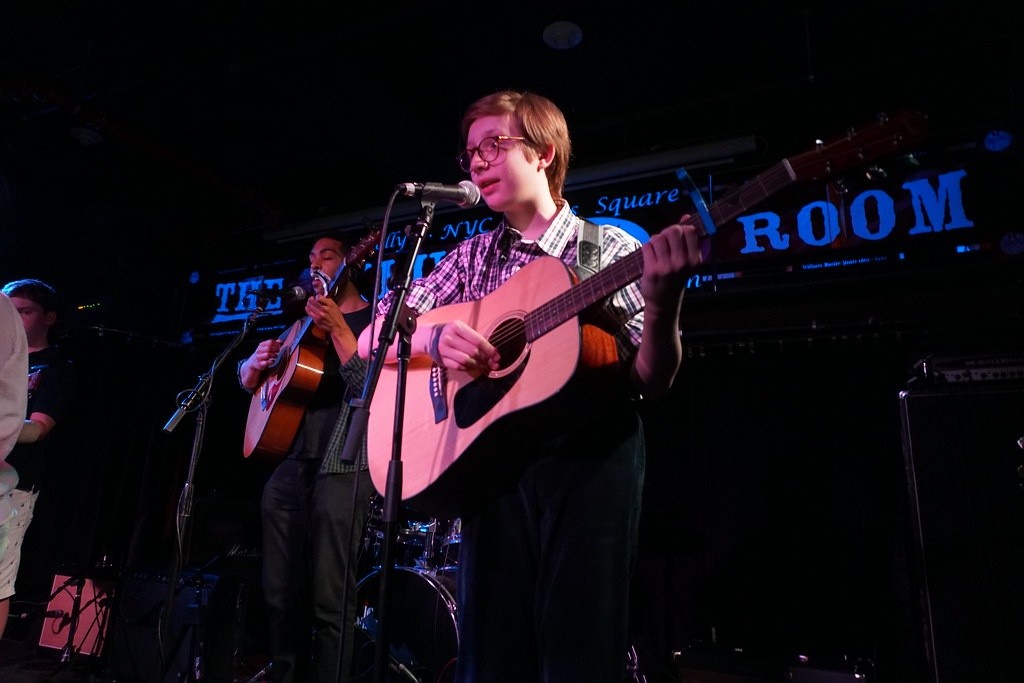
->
[351,517,461,683]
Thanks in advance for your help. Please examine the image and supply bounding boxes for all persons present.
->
[0,278,67,648]
[356,92,708,683]
[219,232,375,683]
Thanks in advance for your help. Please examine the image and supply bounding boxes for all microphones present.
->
[248,286,306,302]
[20,610,63,621]
[396,180,481,208]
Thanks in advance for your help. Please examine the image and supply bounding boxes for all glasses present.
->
[459,134,530,173]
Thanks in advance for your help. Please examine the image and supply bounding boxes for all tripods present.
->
[0,566,97,683]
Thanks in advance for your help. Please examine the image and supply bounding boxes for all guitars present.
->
[242,220,384,462]
[366,110,914,519]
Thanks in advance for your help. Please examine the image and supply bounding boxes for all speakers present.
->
[672,387,1024,683]
[36,567,223,683]
